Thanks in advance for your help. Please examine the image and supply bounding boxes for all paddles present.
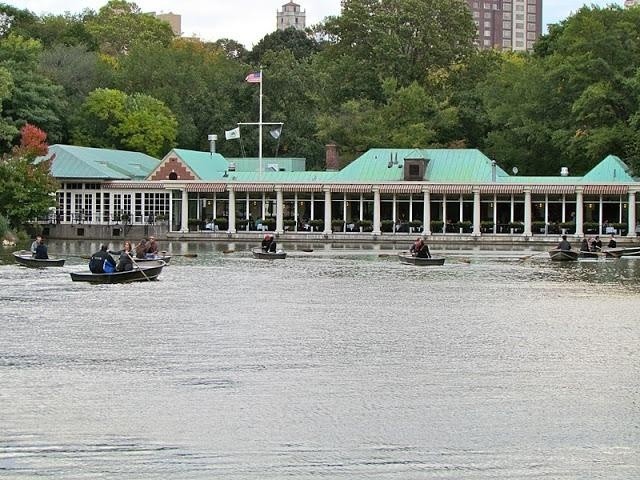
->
[48,253,92,259]
[223,247,262,254]
[520,249,551,261]
[431,251,471,264]
[162,255,197,258]
[276,249,313,252]
[379,254,412,258]
[596,245,621,258]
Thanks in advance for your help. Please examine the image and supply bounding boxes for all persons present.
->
[200,218,208,230]
[30,235,42,257]
[145,235,158,254]
[415,239,431,258]
[608,236,616,248]
[132,239,154,258]
[396,216,402,231]
[34,239,48,259]
[300,214,308,229]
[410,237,422,257]
[106,240,134,271]
[556,235,571,250]
[595,235,602,247]
[265,236,276,253]
[254,216,263,229]
[588,236,601,253]
[88,244,117,274]
[262,234,270,251]
[580,238,588,251]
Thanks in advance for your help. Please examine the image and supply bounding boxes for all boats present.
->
[13,249,65,267]
[548,250,579,260]
[251,250,287,258]
[398,256,445,266]
[581,248,624,259]
[70,255,172,284]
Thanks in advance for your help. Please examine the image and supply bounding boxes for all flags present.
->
[245,72,260,83]
